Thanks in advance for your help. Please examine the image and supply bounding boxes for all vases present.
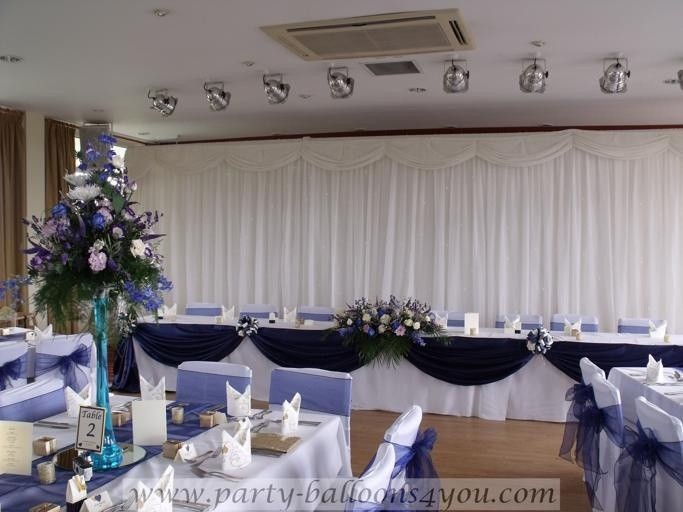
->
[79,283,122,470]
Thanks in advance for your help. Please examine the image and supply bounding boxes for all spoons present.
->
[184,451,213,463]
[248,410,273,419]
[191,467,244,483]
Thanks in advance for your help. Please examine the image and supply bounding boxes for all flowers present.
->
[0,133,173,346]
[0,272,34,309]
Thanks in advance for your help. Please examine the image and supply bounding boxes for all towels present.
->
[220,417,251,471]
[128,465,174,511]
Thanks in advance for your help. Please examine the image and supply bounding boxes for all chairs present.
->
[0,325,96,421]
[559,358,682,512]
[383,406,441,511]
[315,442,395,511]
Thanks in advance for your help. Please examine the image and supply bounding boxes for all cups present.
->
[664,335,671,343]
[470,327,477,336]
[37,461,56,484]
[216,316,224,324]
[576,332,584,341]
[172,407,184,424]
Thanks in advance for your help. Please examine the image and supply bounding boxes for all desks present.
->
[0,314,34,327]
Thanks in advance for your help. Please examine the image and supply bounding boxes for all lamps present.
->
[599,53,632,98]
[261,72,291,105]
[326,65,356,99]
[202,80,232,111]
[518,54,551,95]
[439,56,470,95]
[146,87,177,117]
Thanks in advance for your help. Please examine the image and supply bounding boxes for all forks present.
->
[191,447,221,467]
[251,418,270,432]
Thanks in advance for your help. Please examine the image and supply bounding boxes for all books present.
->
[250,432,302,458]
